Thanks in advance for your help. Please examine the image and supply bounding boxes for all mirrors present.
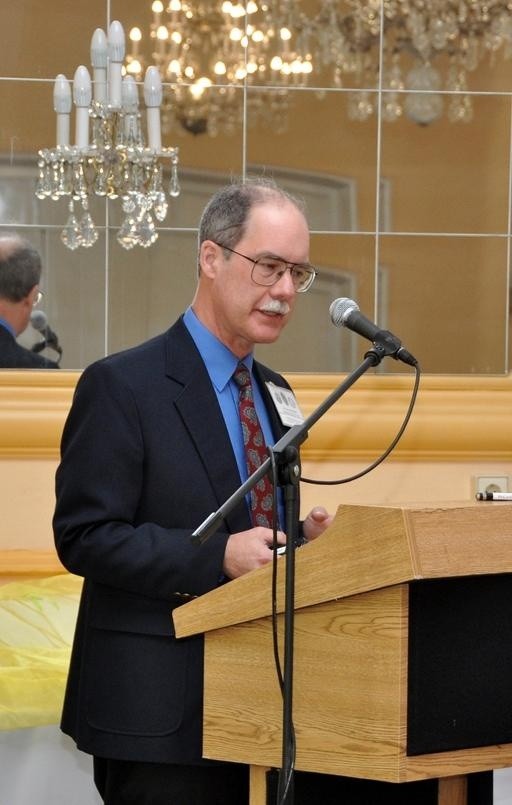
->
[1,1,511,373]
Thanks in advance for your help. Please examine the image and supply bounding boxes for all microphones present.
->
[329,297,418,367]
[31,310,62,353]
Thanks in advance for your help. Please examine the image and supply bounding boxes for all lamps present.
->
[34,14,184,252]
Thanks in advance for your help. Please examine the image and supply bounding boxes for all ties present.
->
[231,363,284,534]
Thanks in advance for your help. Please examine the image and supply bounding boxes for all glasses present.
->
[33,285,44,308]
[215,240,319,294]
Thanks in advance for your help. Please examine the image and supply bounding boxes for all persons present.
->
[0,232,64,372]
[54,179,335,804]
